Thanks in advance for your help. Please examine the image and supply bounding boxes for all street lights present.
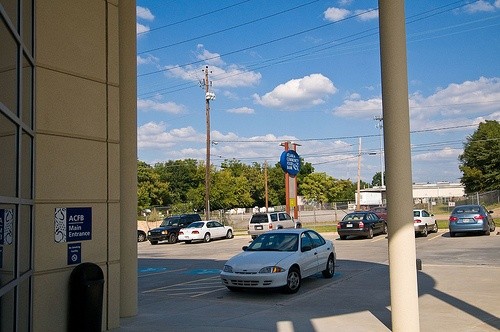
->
[356,137,377,212]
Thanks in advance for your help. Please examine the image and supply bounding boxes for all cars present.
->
[137,230,147,242]
[220,229,336,295]
[413,208,439,237]
[448,204,496,237]
[178,221,234,244]
[336,211,387,240]
[369,207,389,233]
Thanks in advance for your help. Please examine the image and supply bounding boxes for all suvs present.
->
[147,214,204,243]
[247,211,304,242]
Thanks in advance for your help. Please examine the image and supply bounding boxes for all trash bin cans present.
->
[68,262,105,332]
